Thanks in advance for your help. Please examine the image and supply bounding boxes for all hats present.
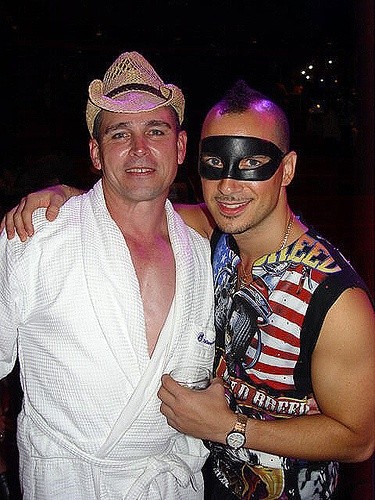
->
[85,51,185,140]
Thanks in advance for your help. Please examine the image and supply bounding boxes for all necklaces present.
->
[279,212,295,250]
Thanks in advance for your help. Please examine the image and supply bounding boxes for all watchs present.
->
[225,414,249,449]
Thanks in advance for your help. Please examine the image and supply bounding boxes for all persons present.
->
[0,52,217,500]
[0,78,375,500]
[315,104,342,146]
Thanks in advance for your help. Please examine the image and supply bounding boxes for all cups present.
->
[169,365,211,390]
